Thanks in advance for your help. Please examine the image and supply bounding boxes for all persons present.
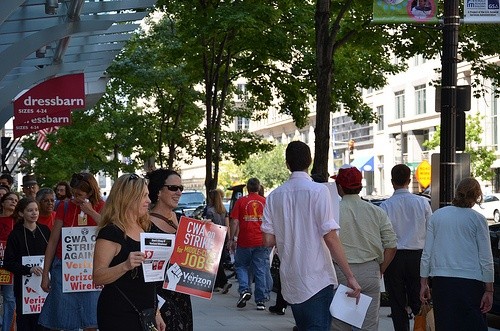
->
[143,169,214,331]
[0,172,107,331]
[329,167,398,331]
[205,177,287,315]
[419,178,494,331]
[378,164,432,331]
[91,174,167,331]
[259,140,362,331]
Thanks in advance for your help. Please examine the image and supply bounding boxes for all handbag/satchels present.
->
[414,298,435,331]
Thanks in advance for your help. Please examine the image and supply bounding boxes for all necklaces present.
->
[168,213,173,219]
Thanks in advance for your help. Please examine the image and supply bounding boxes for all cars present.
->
[471,192,500,223]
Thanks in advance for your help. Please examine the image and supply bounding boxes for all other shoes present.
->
[237,291,251,308]
[221,281,232,294]
[257,301,266,310]
[214,287,221,292]
[269,305,285,315]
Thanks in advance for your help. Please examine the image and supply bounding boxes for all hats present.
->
[330,167,363,189]
[20,175,38,187]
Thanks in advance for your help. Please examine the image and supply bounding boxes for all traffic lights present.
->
[347,141,354,155]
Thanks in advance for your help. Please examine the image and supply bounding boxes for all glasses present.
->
[163,185,183,192]
[5,198,18,203]
[128,174,140,183]
[72,173,85,180]
[42,199,56,203]
[25,186,36,189]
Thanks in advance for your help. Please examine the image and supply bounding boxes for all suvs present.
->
[173,189,207,217]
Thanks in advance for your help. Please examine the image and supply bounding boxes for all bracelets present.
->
[156,311,161,315]
